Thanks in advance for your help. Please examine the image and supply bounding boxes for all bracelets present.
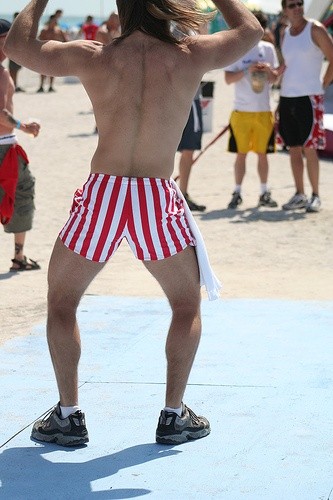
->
[16,120,21,129]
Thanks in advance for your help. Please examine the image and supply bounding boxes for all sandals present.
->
[10,255,40,272]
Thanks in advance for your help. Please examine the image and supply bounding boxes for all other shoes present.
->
[15,88,25,93]
[183,193,206,210]
[49,88,55,91]
[37,88,44,93]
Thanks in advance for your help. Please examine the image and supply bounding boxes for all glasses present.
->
[285,1,303,9]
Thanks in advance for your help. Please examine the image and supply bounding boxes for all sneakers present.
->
[30,401,90,446]
[155,401,211,445]
[228,191,242,209]
[258,192,278,207]
[282,190,309,210]
[306,195,321,211]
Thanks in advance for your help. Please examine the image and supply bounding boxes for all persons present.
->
[178,87,207,211]
[7,9,98,93]
[277,0,333,211]
[95,12,120,133]
[224,10,280,208]
[0,18,42,270]
[2,0,264,446]
